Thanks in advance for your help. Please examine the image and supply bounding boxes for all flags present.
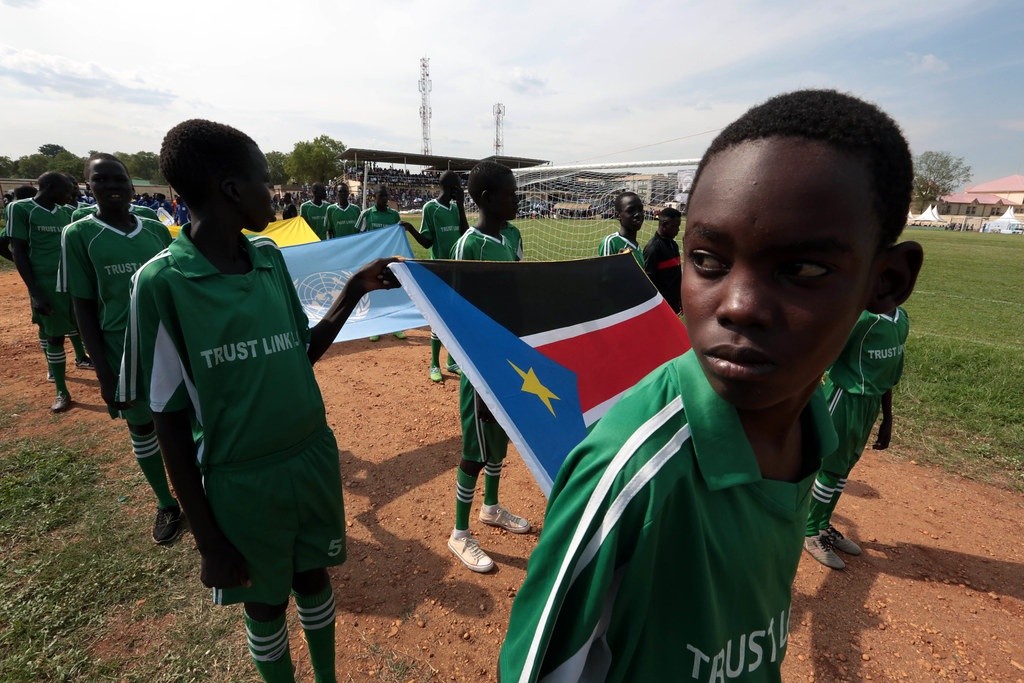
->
[387,253,692,504]
[278,223,430,343]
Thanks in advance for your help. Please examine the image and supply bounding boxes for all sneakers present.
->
[446,363,462,374]
[75,358,96,369]
[47,368,56,383]
[803,532,845,569]
[819,525,861,555]
[369,335,379,341]
[447,533,494,572]
[431,367,442,381]
[393,331,407,339]
[51,393,71,412]
[479,506,530,534]
[152,498,182,543]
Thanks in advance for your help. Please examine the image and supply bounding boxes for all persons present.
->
[111,117,405,683]
[945,222,974,231]
[494,89,912,683]
[597,192,645,272]
[513,171,690,221]
[983,223,1024,235]
[802,240,923,571]
[0,171,400,412]
[399,170,469,381]
[446,161,530,572]
[345,163,479,214]
[54,151,203,544]
[643,209,684,316]
